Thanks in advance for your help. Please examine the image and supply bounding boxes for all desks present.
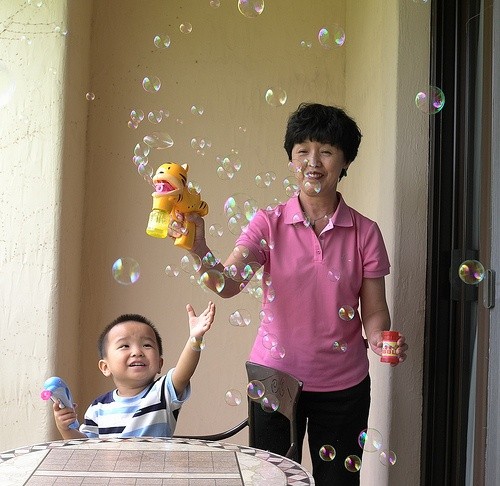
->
[0,435,315,486]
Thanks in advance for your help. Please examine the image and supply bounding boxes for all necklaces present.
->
[304,213,331,230]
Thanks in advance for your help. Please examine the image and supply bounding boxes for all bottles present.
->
[381,331,399,364]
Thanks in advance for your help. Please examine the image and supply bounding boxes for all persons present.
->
[54,300,217,439]
[167,100,408,485]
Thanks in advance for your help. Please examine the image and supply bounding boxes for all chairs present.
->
[172,360,304,459]
